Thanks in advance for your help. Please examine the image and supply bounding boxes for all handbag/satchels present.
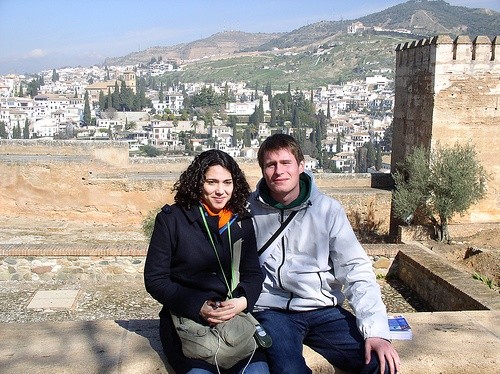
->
[168,298,272,369]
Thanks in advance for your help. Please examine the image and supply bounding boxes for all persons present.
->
[240,134,401,374]
[143,150,269,374]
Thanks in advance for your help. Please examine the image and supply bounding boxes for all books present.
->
[387,315,414,341]
